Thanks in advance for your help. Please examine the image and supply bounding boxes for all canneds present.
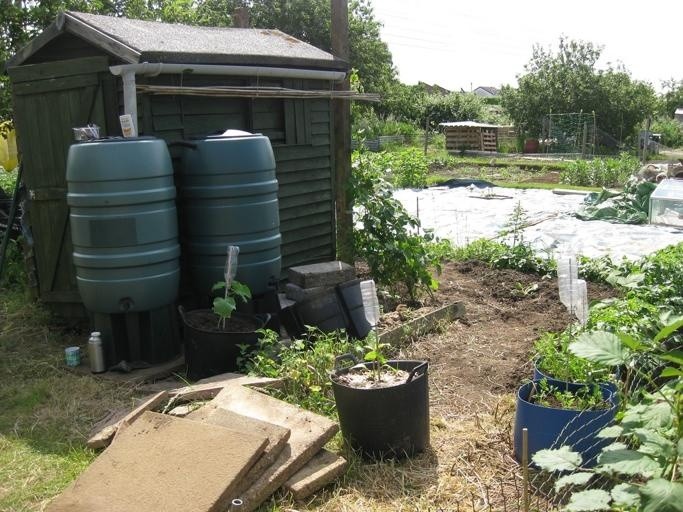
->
[66,347,80,367]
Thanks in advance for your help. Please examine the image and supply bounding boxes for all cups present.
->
[119,111,136,137]
[65,346,81,366]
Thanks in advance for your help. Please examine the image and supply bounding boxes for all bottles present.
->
[88,332,106,373]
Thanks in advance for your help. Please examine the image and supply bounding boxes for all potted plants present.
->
[533,331,620,405]
[177,246,272,381]
[513,378,617,478]
[329,279,429,464]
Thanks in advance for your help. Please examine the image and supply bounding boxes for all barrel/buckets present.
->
[66,138,181,314]
[173,135,282,293]
